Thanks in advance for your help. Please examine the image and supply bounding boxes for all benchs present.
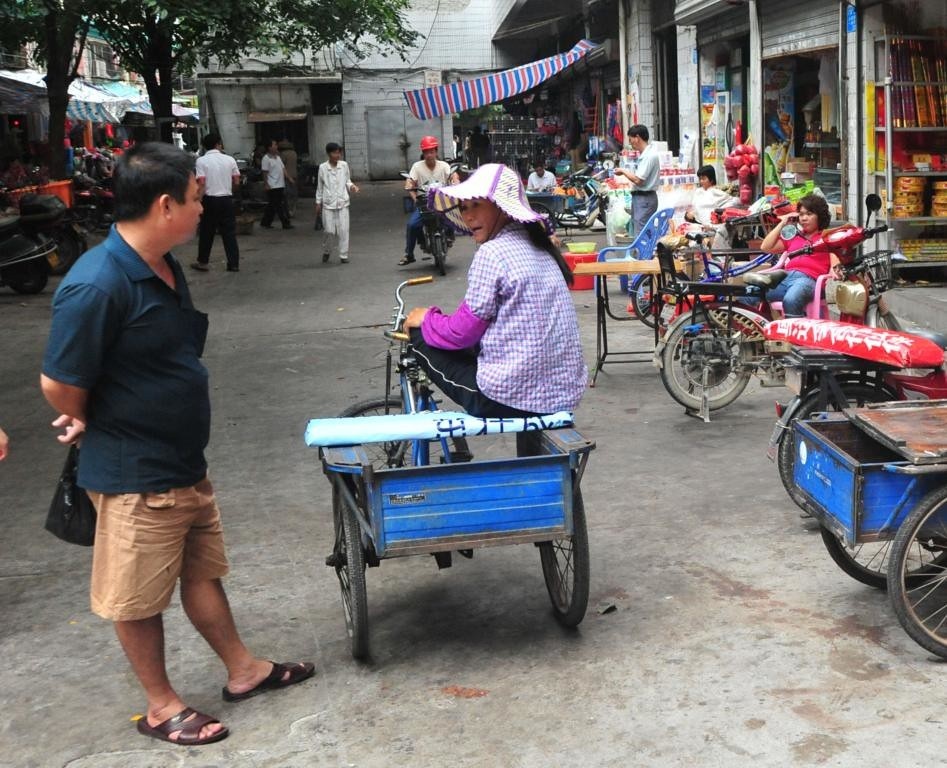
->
[305,410,572,446]
[766,318,945,368]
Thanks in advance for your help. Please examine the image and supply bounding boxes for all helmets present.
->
[419,135,438,151]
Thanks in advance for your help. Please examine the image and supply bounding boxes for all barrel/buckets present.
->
[562,252,600,290]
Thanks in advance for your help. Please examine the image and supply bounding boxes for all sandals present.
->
[398,254,416,266]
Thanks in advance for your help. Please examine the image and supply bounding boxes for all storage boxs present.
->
[781,157,815,187]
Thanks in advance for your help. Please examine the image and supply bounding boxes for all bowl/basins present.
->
[565,242,598,254]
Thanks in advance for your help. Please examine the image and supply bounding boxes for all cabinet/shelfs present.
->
[873,31,947,284]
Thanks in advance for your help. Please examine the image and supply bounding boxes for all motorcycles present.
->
[399,163,461,275]
[0,169,115,295]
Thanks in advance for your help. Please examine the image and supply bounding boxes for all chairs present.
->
[594,208,676,297]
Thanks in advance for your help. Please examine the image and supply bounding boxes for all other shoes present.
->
[282,224,295,230]
[323,253,329,261]
[226,267,240,272]
[341,258,349,263]
[191,263,209,271]
[260,222,273,229]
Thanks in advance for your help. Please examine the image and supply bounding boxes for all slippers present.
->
[222,661,314,704]
[138,707,229,745]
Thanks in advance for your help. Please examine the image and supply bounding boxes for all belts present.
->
[631,191,656,196]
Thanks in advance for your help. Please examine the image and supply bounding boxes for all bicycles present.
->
[630,200,790,329]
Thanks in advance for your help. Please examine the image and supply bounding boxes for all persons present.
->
[612,124,660,260]
[403,164,589,418]
[259,139,301,230]
[684,165,741,235]
[191,134,242,273]
[315,143,360,264]
[397,125,490,266]
[38,139,317,746]
[526,162,557,193]
[734,193,848,319]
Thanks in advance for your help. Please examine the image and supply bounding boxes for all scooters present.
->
[655,196,946,503]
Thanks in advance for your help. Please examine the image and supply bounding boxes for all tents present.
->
[0,68,203,142]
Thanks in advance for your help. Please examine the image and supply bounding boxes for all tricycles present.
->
[792,401,947,658]
[528,167,608,234]
[321,275,597,658]
[234,159,296,218]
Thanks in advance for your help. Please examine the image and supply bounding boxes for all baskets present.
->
[833,249,893,296]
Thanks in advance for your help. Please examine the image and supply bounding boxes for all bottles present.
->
[769,114,792,173]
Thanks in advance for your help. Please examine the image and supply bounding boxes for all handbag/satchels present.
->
[44,445,97,547]
[315,215,322,230]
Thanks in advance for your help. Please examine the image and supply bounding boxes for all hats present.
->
[432,163,549,236]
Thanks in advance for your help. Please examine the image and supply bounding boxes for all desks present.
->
[573,260,683,387]
